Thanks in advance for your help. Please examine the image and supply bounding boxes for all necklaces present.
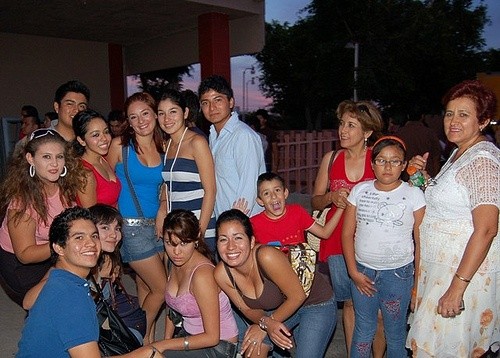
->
[426,134,480,185]
[163,128,188,214]
[231,262,255,310]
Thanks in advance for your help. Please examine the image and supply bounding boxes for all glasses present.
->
[373,158,404,167]
[29,128,60,139]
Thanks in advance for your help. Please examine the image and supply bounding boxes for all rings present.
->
[447,309,454,314]
[247,339,251,343]
[253,342,257,344]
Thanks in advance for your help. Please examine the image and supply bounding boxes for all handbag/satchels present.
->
[86,273,142,358]
[255,242,317,299]
[306,208,332,252]
[104,278,147,339]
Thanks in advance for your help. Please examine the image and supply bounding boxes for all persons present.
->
[407,81,500,358]
[15,205,167,358]
[311,100,386,358]
[142,208,239,358]
[212,209,339,358]
[342,137,426,358]
[0,73,500,358]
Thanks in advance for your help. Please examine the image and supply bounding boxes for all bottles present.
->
[407,166,427,193]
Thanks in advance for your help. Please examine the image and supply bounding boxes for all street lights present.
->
[241,67,256,122]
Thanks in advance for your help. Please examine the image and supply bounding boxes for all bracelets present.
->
[257,315,266,327]
[150,347,157,358]
[184,336,189,351]
[455,273,470,283]
